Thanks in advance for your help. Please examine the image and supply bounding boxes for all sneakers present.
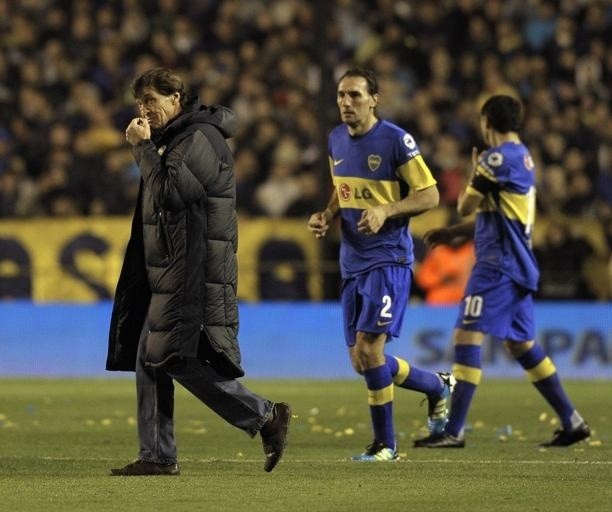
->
[109,455,181,477]
[413,427,466,447]
[348,440,402,461]
[257,399,292,474]
[426,370,455,435]
[536,420,591,447]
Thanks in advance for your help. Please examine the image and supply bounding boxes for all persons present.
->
[105,70,289,477]
[305,66,458,463]
[1,0,611,306]
[414,94,594,448]
